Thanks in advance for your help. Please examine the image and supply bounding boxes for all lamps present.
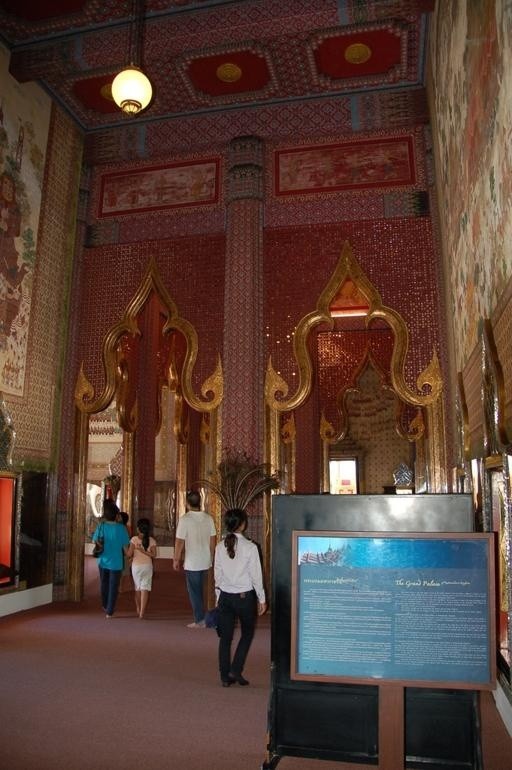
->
[112,0,153,114]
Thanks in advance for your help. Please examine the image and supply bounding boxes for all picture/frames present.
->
[475,449,511,709]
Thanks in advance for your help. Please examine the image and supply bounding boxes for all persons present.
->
[212,507,269,688]
[92,502,130,619]
[171,491,217,630]
[118,511,134,539]
[1,166,25,286]
[125,517,157,619]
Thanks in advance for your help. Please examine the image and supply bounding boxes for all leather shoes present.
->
[222,670,249,689]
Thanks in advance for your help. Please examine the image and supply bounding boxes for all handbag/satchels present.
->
[92,536,104,558]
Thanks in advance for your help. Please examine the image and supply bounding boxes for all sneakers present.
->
[187,622,206,628]
